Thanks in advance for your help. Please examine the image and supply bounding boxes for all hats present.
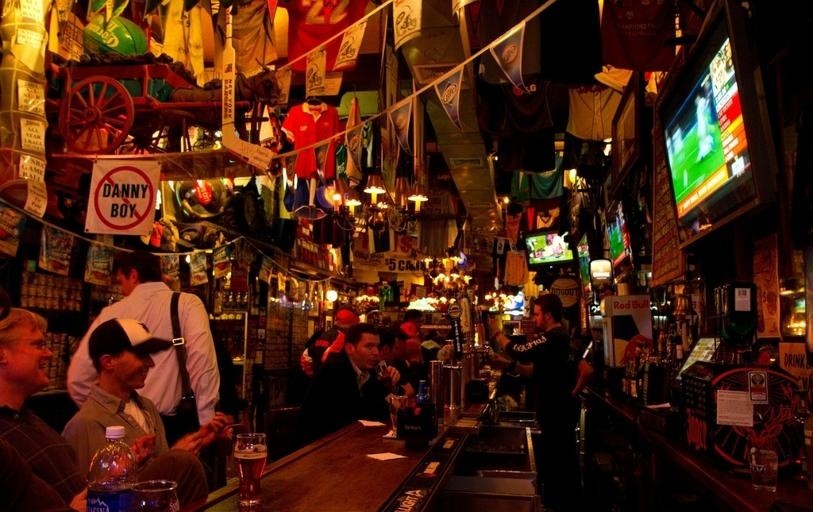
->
[90,318,174,358]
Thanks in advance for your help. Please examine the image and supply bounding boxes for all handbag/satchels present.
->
[175,393,201,433]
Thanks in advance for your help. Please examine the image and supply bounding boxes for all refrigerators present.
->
[599,294,653,369]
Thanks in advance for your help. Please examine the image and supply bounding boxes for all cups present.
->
[232,432,268,505]
[130,475,180,511]
[388,394,408,432]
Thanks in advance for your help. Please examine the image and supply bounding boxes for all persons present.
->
[58,315,230,479]
[0,303,209,511]
[63,249,221,485]
[287,304,424,448]
[485,291,583,511]
[483,293,605,441]
[689,93,716,165]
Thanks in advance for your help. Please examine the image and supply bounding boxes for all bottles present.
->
[224,291,248,310]
[380,359,402,395]
[82,422,138,512]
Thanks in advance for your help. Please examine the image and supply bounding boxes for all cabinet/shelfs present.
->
[575,377,811,511]
[209,309,248,399]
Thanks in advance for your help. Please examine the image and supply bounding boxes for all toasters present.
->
[415,376,430,401]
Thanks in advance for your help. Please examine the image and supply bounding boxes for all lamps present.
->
[333,173,433,213]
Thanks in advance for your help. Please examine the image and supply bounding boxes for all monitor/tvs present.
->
[657,7,758,245]
[502,285,528,317]
[670,334,725,391]
[576,229,595,301]
[605,181,634,279]
[523,227,580,271]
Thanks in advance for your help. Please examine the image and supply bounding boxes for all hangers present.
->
[305,92,323,106]
